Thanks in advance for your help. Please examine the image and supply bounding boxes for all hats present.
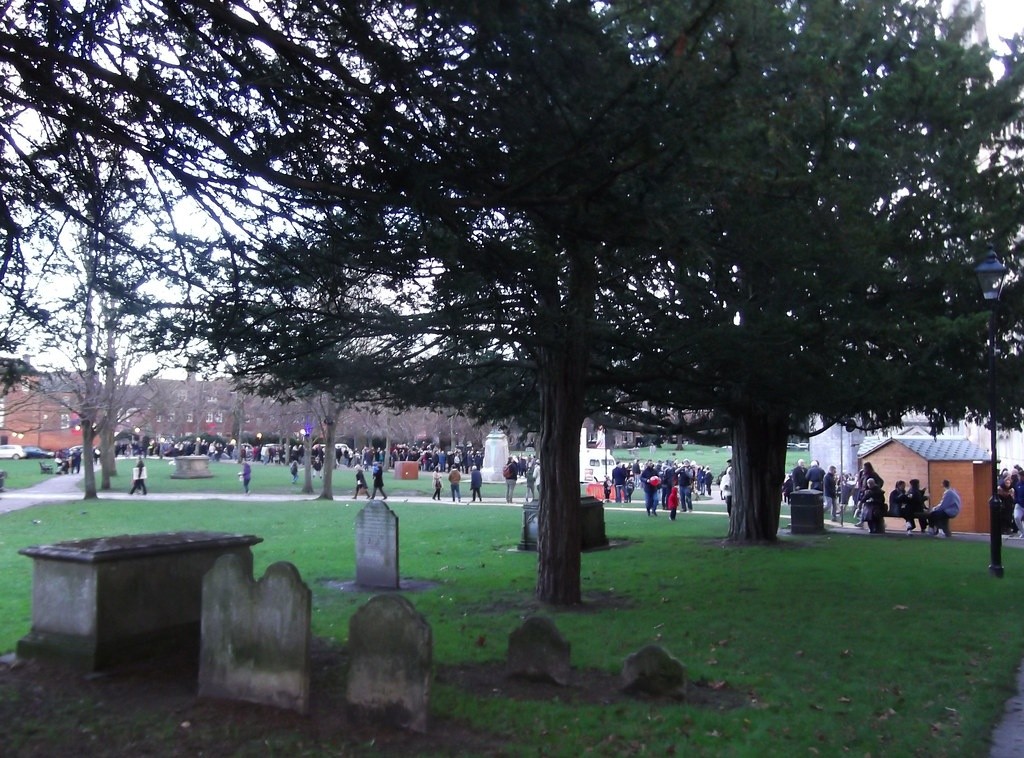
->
[472,466,477,470]
[727,459,732,463]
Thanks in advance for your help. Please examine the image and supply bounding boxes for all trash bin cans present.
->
[790,489,824,536]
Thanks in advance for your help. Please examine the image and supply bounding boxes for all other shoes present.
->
[433,497,436,500]
[352,497,356,499]
[651,511,657,516]
[855,522,863,526]
[366,496,370,499]
[680,509,686,512]
[371,498,373,499]
[648,511,650,516]
[383,496,387,500]
[438,499,441,500]
[689,509,692,512]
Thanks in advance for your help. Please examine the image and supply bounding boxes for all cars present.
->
[23,446,55,459]
[786,442,809,451]
[69,446,84,457]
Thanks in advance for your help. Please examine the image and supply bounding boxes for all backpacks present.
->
[503,463,514,478]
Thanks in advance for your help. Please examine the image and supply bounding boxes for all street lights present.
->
[973,250,1006,578]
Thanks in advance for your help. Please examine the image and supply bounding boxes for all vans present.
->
[0,445,24,460]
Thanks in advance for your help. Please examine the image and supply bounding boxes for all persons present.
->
[611,460,712,520]
[433,466,442,500]
[372,462,379,479]
[311,444,324,478]
[114,438,237,462]
[352,467,370,499]
[717,459,732,518]
[336,446,386,471]
[54,446,99,474]
[470,466,482,501]
[997,465,1024,537]
[782,459,885,533]
[506,454,540,503]
[889,479,960,536]
[128,456,147,495]
[371,462,387,499]
[242,444,262,461]
[264,444,305,484]
[390,442,484,474]
[449,466,461,502]
[238,462,251,496]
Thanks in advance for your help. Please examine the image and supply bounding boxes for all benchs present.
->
[866,511,951,537]
[40,462,53,474]
